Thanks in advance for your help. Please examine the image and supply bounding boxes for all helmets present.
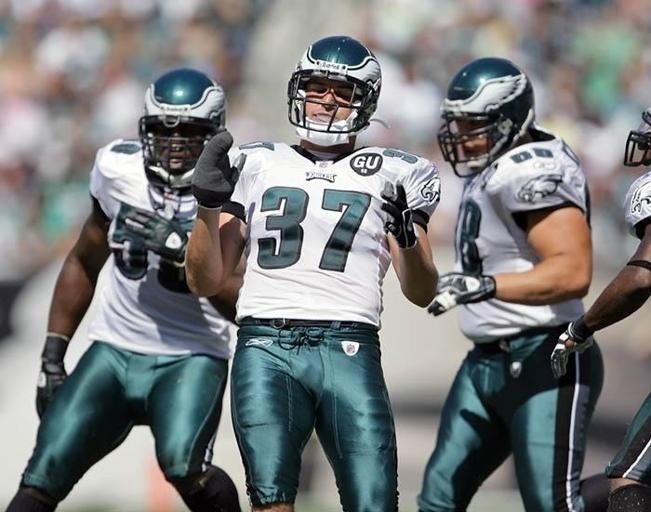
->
[437,58,535,178]
[138,69,226,191]
[624,107,651,166]
[287,36,381,147]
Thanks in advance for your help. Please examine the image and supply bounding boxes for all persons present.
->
[4,63,248,512]
[407,52,607,512]
[181,30,445,512]
[547,100,651,512]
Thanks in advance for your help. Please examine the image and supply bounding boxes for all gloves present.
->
[379,180,419,251]
[549,323,593,377]
[37,361,68,418]
[120,209,188,269]
[193,131,246,207]
[428,273,496,316]
